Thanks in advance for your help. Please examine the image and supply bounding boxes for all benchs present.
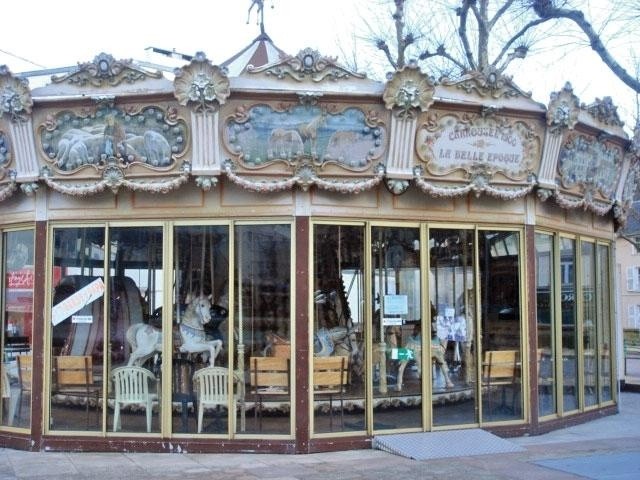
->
[16,354,102,431]
[482,350,542,416]
[249,357,349,432]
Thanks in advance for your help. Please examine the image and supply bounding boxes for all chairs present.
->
[115,358,241,433]
